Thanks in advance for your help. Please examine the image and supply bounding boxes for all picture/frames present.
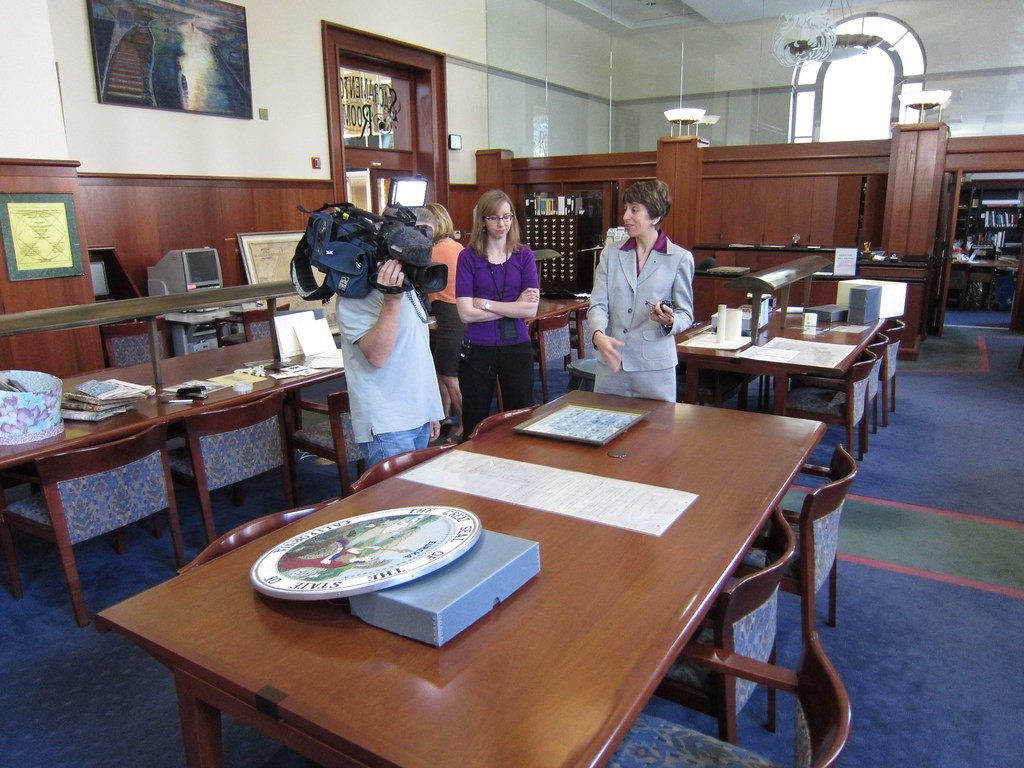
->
[0,193,85,282]
[236,229,341,336]
[87,0,254,121]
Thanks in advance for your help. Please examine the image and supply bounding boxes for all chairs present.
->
[732,443,859,646]
[99,317,170,369]
[347,439,458,493]
[282,391,364,498]
[651,503,797,744]
[241,304,291,342]
[970,246,996,260]
[867,332,889,436]
[885,318,907,412]
[987,264,1018,312]
[174,497,341,574]
[530,309,573,404]
[0,420,184,629]
[577,307,595,359]
[215,310,247,348]
[150,389,297,548]
[466,404,540,440]
[948,263,971,312]
[600,630,854,768]
[782,347,877,462]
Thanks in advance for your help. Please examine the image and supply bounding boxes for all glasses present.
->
[483,212,514,223]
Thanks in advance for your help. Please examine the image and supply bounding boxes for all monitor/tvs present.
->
[147,248,223,293]
[88,261,110,296]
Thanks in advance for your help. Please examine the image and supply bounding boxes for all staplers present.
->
[176,386,208,399]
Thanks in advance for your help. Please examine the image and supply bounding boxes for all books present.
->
[61,378,151,421]
[998,255,1016,260]
[525,192,582,215]
[978,210,1013,246]
[606,227,629,245]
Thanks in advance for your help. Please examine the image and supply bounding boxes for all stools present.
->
[564,359,595,393]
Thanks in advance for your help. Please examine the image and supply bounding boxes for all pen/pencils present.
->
[161,399,193,403]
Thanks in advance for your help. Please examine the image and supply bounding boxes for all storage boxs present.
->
[847,286,881,325]
[836,278,908,319]
[349,528,543,648]
[748,296,777,309]
[804,303,848,323]
[711,312,752,330]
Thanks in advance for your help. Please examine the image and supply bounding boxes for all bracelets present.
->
[664,326,672,327]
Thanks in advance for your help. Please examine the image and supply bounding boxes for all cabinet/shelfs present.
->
[694,243,928,284]
[972,179,1024,255]
[953,182,985,249]
[525,213,592,300]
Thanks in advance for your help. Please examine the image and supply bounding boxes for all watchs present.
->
[485,300,490,310]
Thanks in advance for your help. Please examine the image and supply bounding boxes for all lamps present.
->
[664,108,706,137]
[783,0,885,61]
[932,98,953,123]
[898,89,952,122]
[691,115,721,137]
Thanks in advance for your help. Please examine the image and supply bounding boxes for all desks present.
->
[0,328,346,605]
[90,388,827,768]
[674,305,888,416]
[428,296,590,347]
[950,259,1019,310]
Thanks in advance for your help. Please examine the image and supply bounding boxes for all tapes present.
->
[233,382,253,391]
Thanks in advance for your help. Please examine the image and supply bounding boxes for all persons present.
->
[335,207,445,468]
[586,180,694,405]
[422,204,465,443]
[699,257,716,270]
[455,189,540,443]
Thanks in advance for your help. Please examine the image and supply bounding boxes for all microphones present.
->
[387,226,432,266]
